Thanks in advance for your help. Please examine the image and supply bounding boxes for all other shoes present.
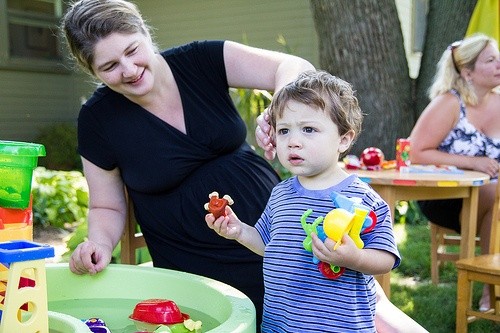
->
[479,288,492,311]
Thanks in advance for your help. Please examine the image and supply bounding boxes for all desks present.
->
[337,160,490,310]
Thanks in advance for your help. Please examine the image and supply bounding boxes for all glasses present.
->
[449,39,463,75]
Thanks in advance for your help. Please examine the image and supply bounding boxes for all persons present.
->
[60,1,432,333]
[407,34,500,317]
[203,70,402,333]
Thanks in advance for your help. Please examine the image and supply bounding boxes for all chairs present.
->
[429,221,482,286]
[121,184,148,265]
[455,171,500,333]
